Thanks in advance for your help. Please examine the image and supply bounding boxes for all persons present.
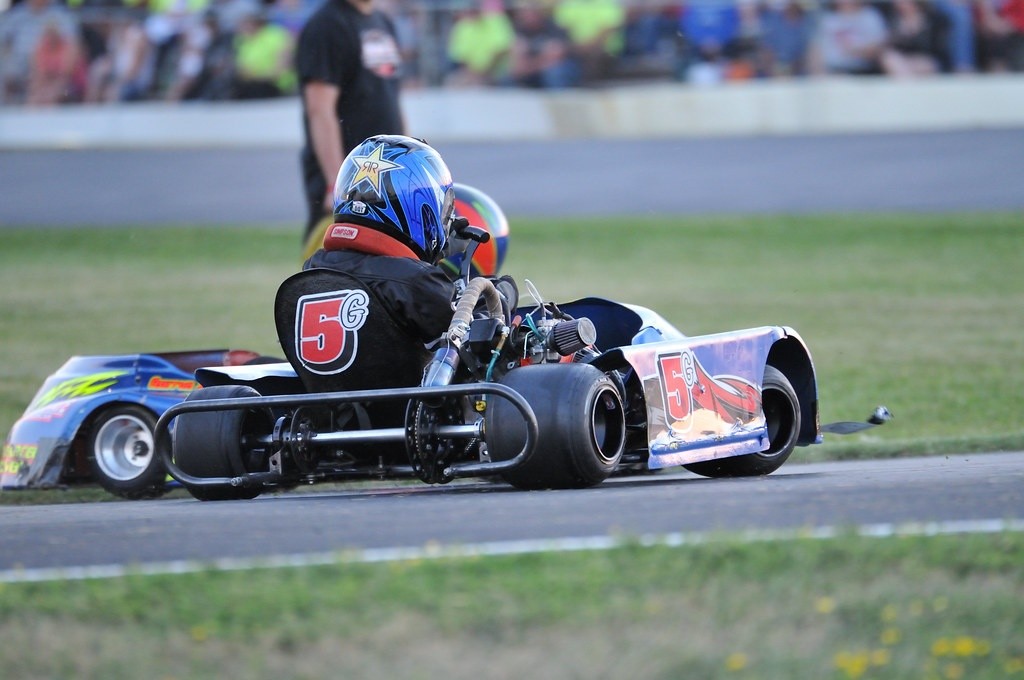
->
[301,0,406,247]
[384,0,1024,88]
[301,135,593,365]
[0,0,324,105]
